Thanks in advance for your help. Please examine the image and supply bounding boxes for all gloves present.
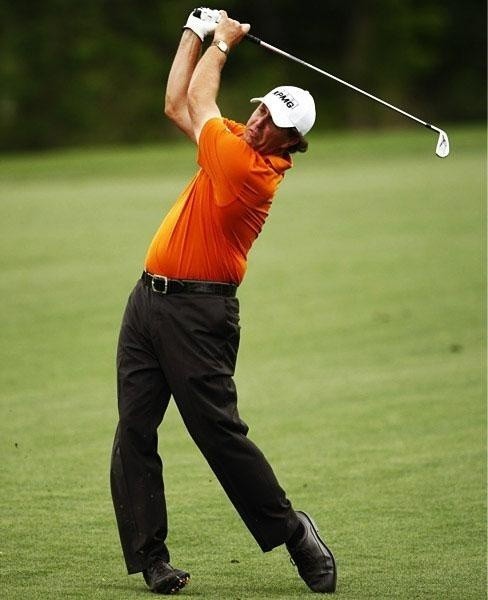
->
[184,7,227,42]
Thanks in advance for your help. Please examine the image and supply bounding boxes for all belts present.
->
[141,270,236,296]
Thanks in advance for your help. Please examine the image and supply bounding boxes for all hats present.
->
[250,86,316,136]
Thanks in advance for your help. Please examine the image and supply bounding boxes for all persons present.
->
[106,7,335,593]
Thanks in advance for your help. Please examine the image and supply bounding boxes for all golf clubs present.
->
[215,14,449,158]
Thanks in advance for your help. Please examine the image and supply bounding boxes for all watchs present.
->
[210,39,228,53]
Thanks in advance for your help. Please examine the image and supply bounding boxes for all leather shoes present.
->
[143,561,190,594]
[285,511,336,593]
